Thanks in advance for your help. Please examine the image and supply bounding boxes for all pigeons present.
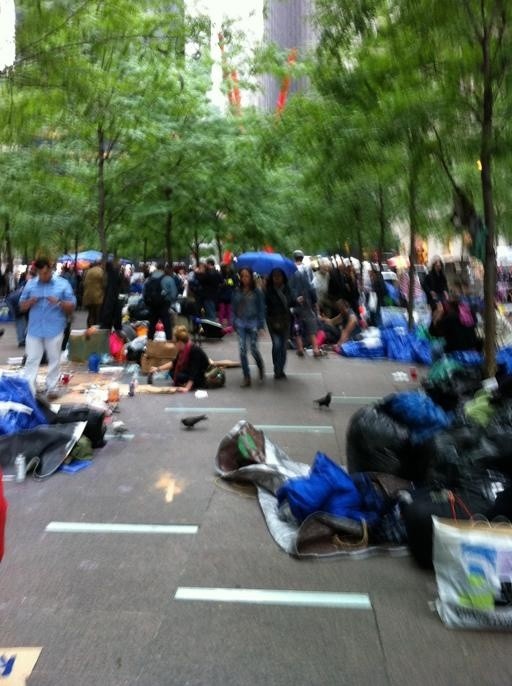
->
[112,419,129,440]
[312,391,333,409]
[179,413,208,430]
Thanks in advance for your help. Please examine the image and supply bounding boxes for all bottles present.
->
[13,453,26,480]
[108,379,119,401]
[155,319,164,332]
[88,352,99,374]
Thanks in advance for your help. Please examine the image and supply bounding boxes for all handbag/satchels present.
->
[426,509,512,631]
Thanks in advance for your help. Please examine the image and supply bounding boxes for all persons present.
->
[147,326,210,391]
[396,254,511,355]
[6,253,241,361]
[231,267,305,388]
[287,249,387,358]
[19,255,78,395]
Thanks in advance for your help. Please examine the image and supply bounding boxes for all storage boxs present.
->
[67,329,109,362]
[140,341,178,375]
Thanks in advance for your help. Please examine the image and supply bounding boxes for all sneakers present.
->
[258,369,286,385]
[239,374,251,388]
[45,386,61,400]
[296,346,329,358]
